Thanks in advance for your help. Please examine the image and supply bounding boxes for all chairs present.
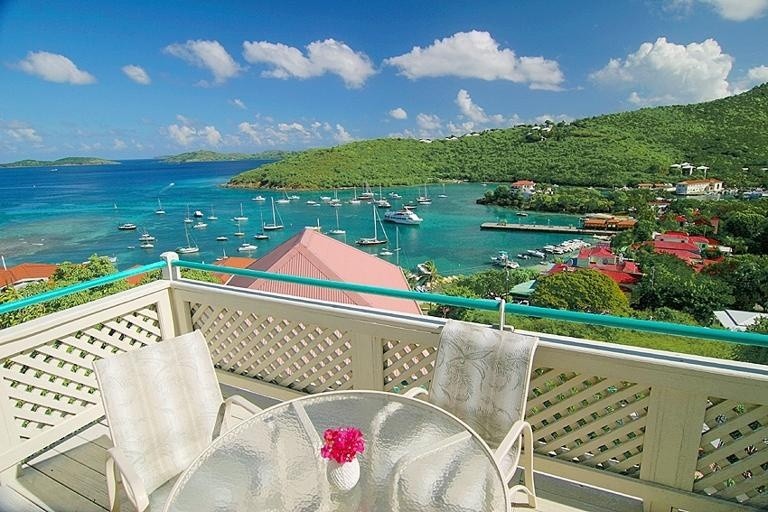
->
[91,329,280,512]
[368,318,540,512]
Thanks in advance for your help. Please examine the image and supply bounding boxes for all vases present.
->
[327,456,362,489]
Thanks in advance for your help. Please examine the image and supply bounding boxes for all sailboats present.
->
[263,195,285,230]
[253,211,269,239]
[234,202,248,221]
[355,204,390,246]
[175,221,200,254]
[234,217,244,236]
[328,209,346,235]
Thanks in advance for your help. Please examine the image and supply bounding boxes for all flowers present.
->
[320,425,366,462]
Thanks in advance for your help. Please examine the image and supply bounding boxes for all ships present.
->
[384,207,423,226]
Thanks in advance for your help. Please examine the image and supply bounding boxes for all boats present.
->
[488,235,610,267]
[416,182,431,205]
[118,223,136,230]
[216,236,229,241]
[346,181,402,208]
[208,215,218,220]
[138,234,155,241]
[193,210,204,217]
[287,195,301,200]
[193,221,208,228]
[275,198,291,204]
[156,209,165,214]
[251,195,265,201]
[306,195,343,207]
[237,243,258,252]
[183,204,194,223]
[140,243,155,248]
[304,225,322,231]
